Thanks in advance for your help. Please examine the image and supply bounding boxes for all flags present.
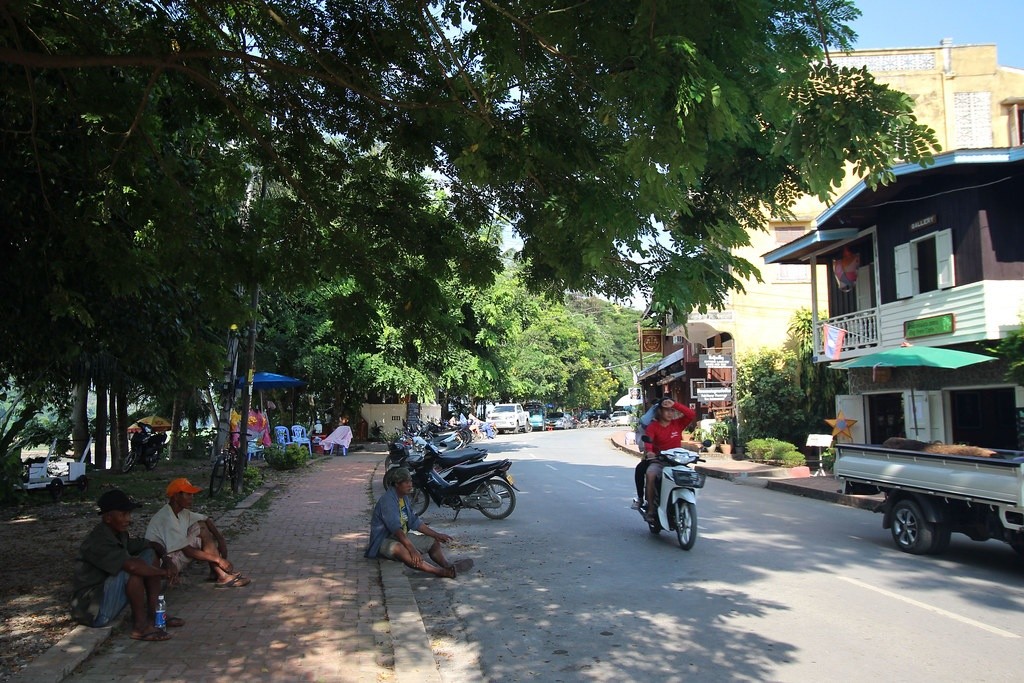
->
[822,323,846,360]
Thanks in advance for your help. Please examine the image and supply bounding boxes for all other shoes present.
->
[631,498,644,509]
[646,511,655,523]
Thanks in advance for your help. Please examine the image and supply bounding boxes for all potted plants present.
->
[732,433,746,454]
[712,420,736,454]
[681,428,716,453]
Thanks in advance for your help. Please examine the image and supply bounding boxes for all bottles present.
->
[154,595,167,634]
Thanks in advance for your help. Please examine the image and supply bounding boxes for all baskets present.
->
[672,469,706,488]
[405,455,422,470]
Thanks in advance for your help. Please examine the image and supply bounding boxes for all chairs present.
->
[624,432,636,445]
[248,439,263,462]
[319,426,354,456]
[275,426,296,452]
[291,425,312,455]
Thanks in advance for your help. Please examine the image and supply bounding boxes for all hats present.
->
[166,478,203,496]
[392,468,412,483]
[97,490,138,515]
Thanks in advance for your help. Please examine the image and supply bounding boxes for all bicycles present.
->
[209,427,252,497]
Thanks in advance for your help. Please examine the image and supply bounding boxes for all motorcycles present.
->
[383,417,488,491]
[395,442,520,522]
[633,435,711,549]
[121,420,167,474]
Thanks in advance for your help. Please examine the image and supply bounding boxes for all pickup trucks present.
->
[487,403,531,434]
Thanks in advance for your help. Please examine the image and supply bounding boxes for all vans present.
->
[524,403,545,431]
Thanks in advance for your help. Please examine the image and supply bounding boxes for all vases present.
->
[362,444,388,452]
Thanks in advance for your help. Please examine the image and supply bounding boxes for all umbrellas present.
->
[827,341,999,440]
[127,413,172,433]
[236,372,306,412]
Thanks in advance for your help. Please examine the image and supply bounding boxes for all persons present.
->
[363,468,474,579]
[630,398,664,511]
[66,488,186,640]
[643,396,696,522]
[145,478,252,590]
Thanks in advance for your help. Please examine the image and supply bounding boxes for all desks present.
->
[310,434,328,456]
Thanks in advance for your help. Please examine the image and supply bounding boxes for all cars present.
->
[610,411,630,426]
[547,412,574,429]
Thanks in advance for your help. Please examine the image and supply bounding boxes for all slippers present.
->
[166,617,183,626]
[453,559,473,572]
[130,628,169,641]
[449,565,456,578]
[216,576,242,587]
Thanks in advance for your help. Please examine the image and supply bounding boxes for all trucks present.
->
[833,444,1024,554]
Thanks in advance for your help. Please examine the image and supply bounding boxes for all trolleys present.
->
[23,438,92,500]
